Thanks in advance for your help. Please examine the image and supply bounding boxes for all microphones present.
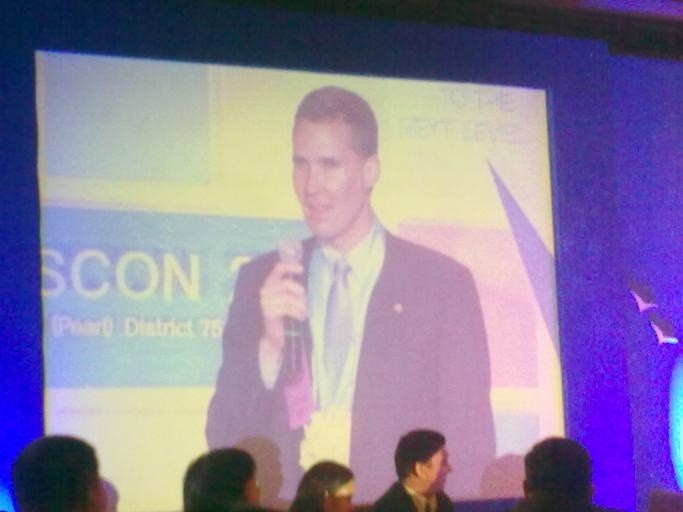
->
[277,235,306,379]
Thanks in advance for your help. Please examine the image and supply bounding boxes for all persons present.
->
[370,430,454,512]
[205,86,496,506]
[509,438,609,512]
[290,461,354,512]
[183,448,263,512]
[13,435,107,512]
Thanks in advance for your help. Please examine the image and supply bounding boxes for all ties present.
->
[322,258,353,395]
[425,503,432,512]
